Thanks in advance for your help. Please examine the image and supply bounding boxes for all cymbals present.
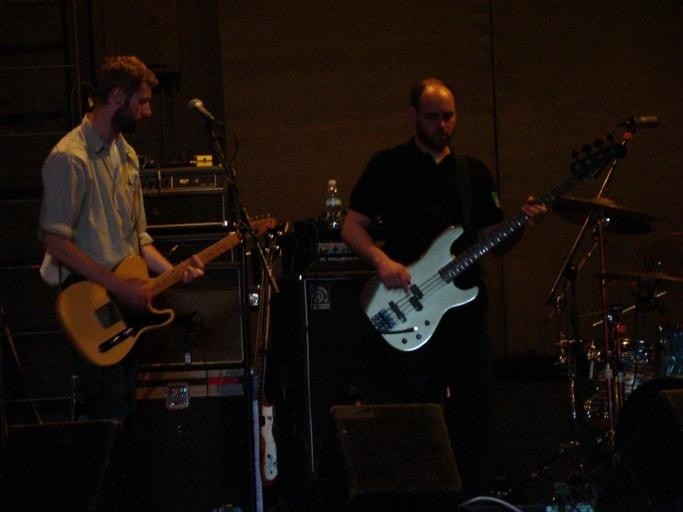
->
[597,273,683,284]
[551,196,660,220]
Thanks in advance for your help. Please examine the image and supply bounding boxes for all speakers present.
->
[129,236,249,371]
[323,404,463,500]
[276,279,381,480]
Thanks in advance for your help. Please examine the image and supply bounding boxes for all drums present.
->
[615,365,660,398]
[614,338,663,374]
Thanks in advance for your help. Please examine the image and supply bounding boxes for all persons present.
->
[342,78,549,446]
[36,54,206,504]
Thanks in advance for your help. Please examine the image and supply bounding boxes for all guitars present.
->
[255,220,288,482]
[360,133,629,353]
[53,213,276,372]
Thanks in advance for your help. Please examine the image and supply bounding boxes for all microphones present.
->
[189,99,221,127]
[630,115,660,125]
